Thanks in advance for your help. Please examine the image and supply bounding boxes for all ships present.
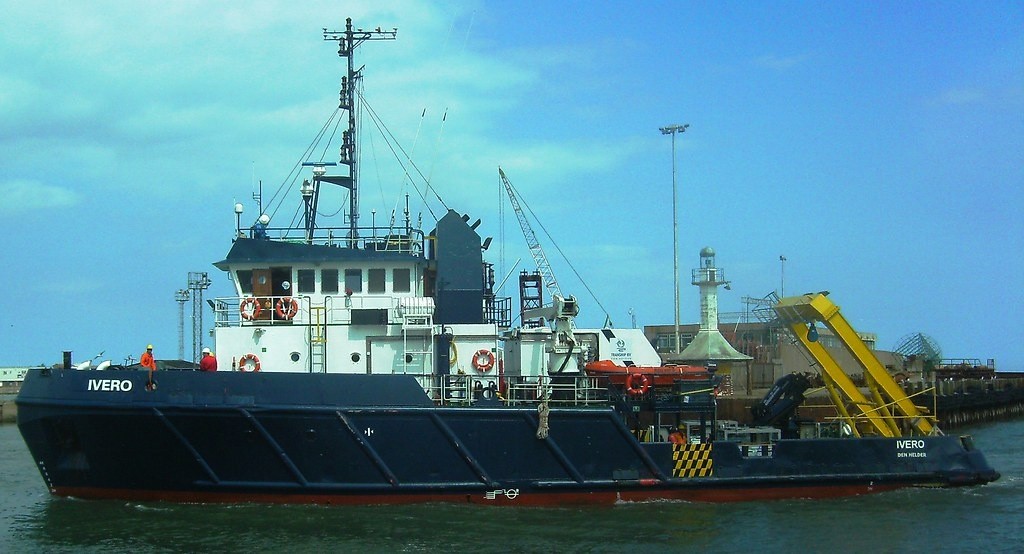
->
[14,15,1002,508]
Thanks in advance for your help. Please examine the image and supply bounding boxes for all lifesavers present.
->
[241,297,261,320]
[625,373,650,395]
[275,297,298,320]
[239,354,260,374]
[473,349,494,371]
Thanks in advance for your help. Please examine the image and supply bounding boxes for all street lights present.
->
[657,122,690,353]
[779,255,787,300]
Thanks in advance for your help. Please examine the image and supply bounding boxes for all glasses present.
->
[147,349,152,350]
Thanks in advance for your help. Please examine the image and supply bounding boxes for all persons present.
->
[200,347,217,372]
[141,344,157,391]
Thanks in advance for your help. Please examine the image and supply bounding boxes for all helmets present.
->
[209,352,214,356]
[202,348,210,353]
[146,345,153,349]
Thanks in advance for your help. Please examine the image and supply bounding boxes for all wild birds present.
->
[93,350,105,359]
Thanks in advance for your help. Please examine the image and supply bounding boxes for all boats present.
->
[585,359,709,388]
